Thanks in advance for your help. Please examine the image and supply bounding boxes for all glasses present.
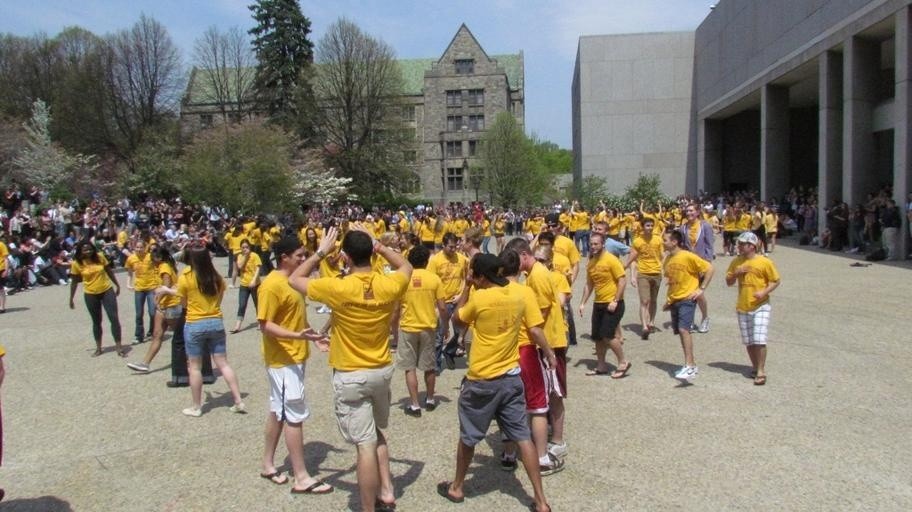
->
[548,225,557,228]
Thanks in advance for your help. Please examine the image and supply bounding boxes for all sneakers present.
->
[546,441,568,458]
[501,450,517,471]
[231,402,245,412]
[642,324,654,339]
[132,334,152,344]
[182,409,201,417]
[404,405,421,417]
[538,452,566,475]
[59,278,72,284]
[127,363,149,371]
[689,318,710,333]
[675,364,698,379]
[167,382,189,386]
[442,331,465,369]
[425,396,436,411]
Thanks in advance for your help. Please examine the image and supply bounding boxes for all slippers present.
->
[751,370,766,384]
[437,481,464,503]
[291,480,334,494]
[585,368,608,375]
[375,495,396,509]
[532,501,551,512]
[612,363,631,378]
[260,470,288,484]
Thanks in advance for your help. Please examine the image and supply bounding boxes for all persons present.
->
[0,331,5,497]
[0,185,248,298]
[713,189,798,386]
[67,236,246,415]
[787,184,901,260]
[228,196,715,512]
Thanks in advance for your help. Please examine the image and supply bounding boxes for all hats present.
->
[545,214,557,223]
[472,254,509,286]
[733,231,758,245]
